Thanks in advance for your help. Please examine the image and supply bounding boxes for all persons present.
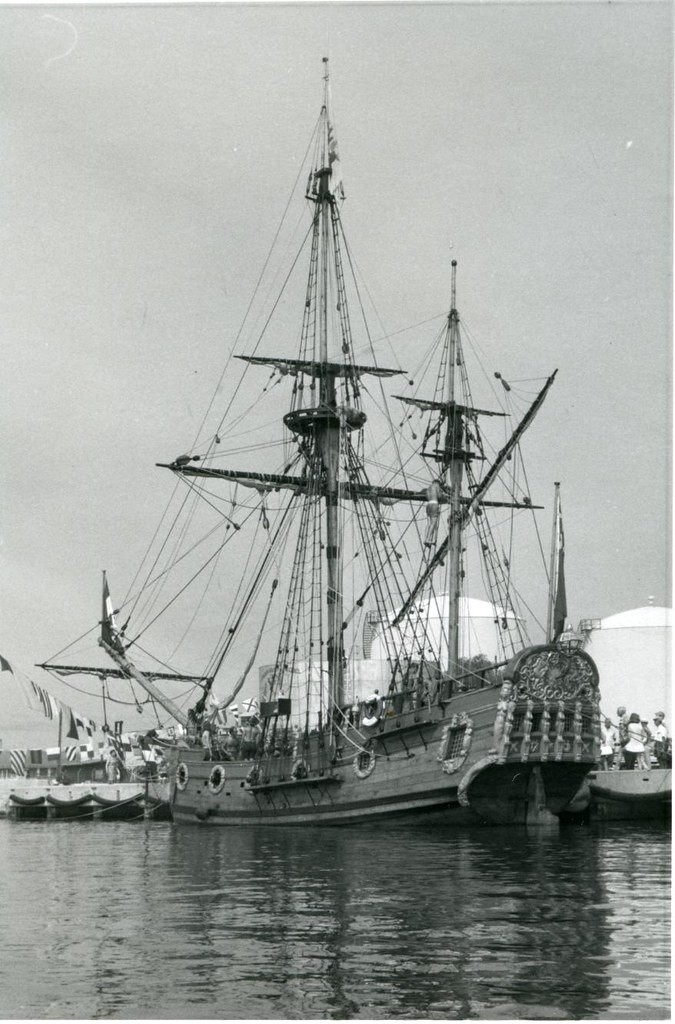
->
[365,689,381,700]
[105,749,121,784]
[600,707,669,770]
[201,715,261,762]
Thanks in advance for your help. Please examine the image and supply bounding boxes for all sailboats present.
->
[27,50,609,833]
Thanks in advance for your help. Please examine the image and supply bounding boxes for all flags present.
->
[0,570,261,781]
